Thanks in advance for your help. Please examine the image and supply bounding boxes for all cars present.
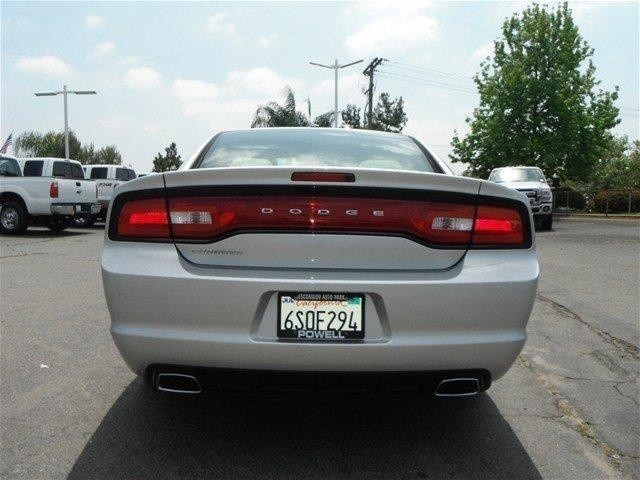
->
[99,126,542,408]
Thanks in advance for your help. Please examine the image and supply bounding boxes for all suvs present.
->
[485,164,561,232]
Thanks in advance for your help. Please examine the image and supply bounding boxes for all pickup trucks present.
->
[0,152,155,234]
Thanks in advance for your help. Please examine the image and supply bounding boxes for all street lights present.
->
[310,57,364,127]
[33,84,96,159]
